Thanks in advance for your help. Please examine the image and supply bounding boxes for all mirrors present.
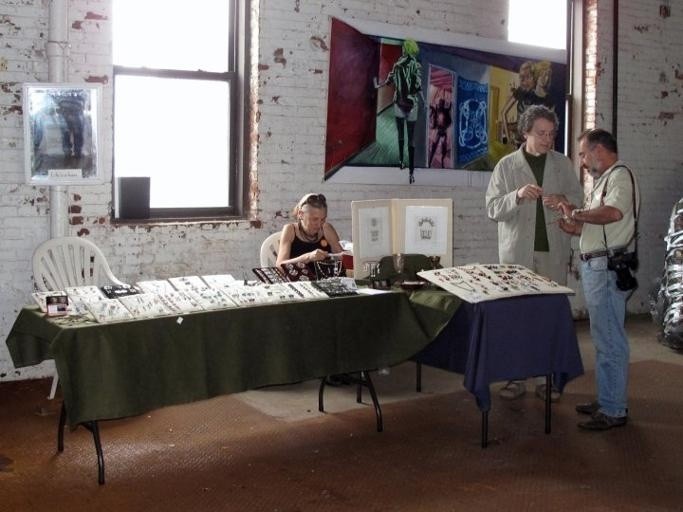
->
[21,81,103,186]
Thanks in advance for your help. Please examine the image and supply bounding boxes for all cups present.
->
[392,252,405,274]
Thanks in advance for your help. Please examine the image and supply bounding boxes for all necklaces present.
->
[297,220,319,242]
[159,293,181,317]
[460,266,485,277]
[137,276,169,298]
[194,288,227,313]
[451,281,476,292]
[316,260,342,278]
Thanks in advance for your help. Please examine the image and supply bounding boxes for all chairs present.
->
[378,254,434,283]
[259,231,280,282]
[31,236,124,400]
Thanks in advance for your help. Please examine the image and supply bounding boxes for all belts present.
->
[579,248,628,261]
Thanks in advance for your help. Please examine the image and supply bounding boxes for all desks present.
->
[408,293,584,449]
[4,279,408,485]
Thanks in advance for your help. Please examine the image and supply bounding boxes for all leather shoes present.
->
[324,374,341,386]
[577,412,627,430]
[339,375,350,385]
[576,401,628,415]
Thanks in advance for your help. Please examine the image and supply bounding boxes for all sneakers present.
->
[536,384,559,403]
[500,380,525,398]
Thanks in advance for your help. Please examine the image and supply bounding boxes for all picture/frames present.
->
[391,196,454,276]
[350,199,390,281]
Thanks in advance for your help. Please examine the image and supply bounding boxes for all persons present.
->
[428,97,453,169]
[522,61,563,151]
[555,126,640,433]
[483,105,585,402]
[497,60,536,142]
[46,90,91,164]
[371,40,430,186]
[275,193,345,269]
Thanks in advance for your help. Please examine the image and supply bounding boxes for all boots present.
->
[408,146,415,184]
[399,139,405,169]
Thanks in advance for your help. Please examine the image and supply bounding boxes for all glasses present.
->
[300,195,325,208]
